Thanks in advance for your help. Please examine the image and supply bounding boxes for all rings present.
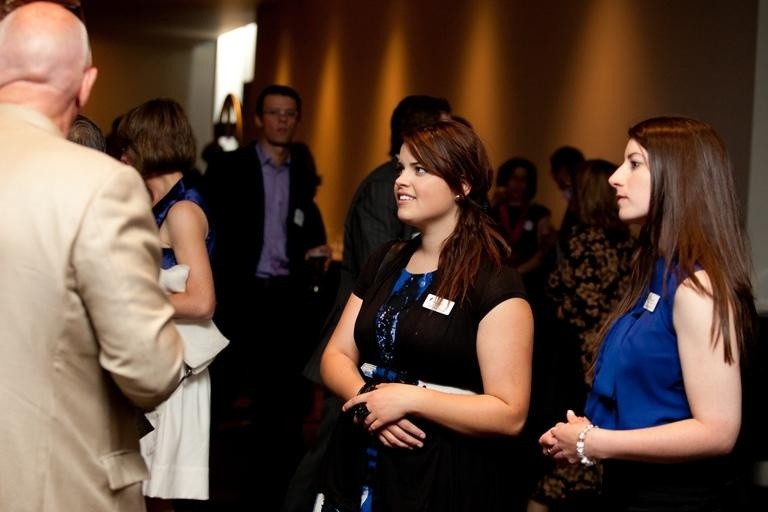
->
[354,404,368,420]
[547,447,553,455]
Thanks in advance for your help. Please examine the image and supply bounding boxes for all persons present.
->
[546,157,635,374]
[312,119,535,512]
[335,93,474,316]
[116,97,231,512]
[203,84,332,512]
[525,113,756,512]
[0,0,186,512]
[549,144,586,268]
[67,112,122,169]
[490,155,551,275]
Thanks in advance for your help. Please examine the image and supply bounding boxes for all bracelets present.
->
[575,423,599,467]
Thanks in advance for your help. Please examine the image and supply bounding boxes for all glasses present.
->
[265,106,299,119]
